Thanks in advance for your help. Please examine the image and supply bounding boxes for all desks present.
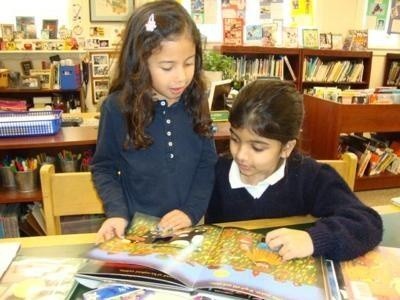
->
[0,205,399,299]
[212,120,232,141]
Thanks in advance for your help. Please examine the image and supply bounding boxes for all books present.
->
[82,287,236,300]
[208,54,400,177]
[0,255,87,300]
[73,212,342,300]
[1,204,46,238]
[337,246,400,300]
[0,244,21,274]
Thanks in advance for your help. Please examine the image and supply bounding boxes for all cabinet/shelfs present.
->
[0,50,89,112]
[300,88,400,191]
[300,49,372,95]
[213,45,301,93]
[0,125,98,206]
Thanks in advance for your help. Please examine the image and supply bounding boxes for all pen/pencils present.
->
[36,152,51,163]
[10,156,38,173]
[58,148,81,160]
[0,155,12,168]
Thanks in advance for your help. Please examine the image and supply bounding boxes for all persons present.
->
[204,79,383,262]
[88,1,219,246]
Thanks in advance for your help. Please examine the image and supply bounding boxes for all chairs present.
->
[40,163,105,237]
[316,151,358,195]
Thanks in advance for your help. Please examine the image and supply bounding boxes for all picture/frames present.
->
[89,0,135,22]
[91,78,110,104]
[1,25,14,40]
[90,52,110,79]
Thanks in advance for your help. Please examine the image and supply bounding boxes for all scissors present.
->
[82,150,93,165]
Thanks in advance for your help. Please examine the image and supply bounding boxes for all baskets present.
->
[0,110,64,138]
